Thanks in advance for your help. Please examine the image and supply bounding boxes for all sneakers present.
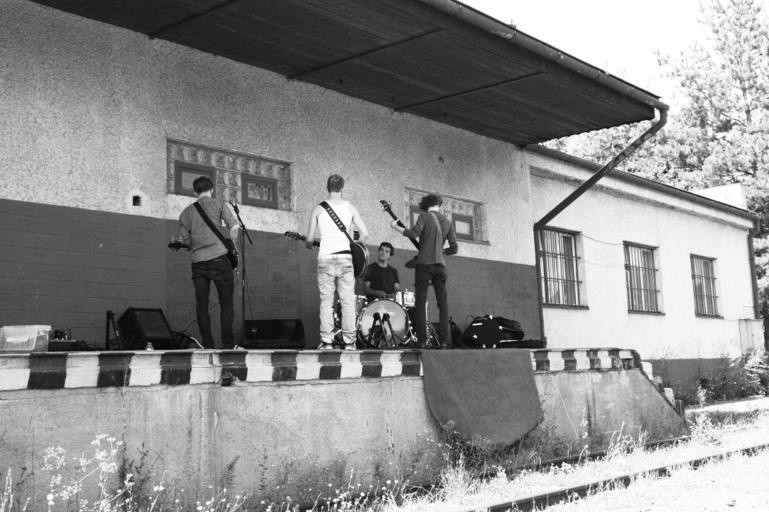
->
[415,343,453,349]
[317,344,356,351]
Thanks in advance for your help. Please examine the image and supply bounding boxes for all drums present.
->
[394,291,416,307]
[334,295,368,331]
[357,298,411,349]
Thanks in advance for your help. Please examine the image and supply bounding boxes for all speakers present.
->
[244,318,305,348]
[462,315,524,347]
[119,308,176,349]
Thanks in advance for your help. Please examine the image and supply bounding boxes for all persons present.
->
[305,174,369,351]
[363,242,401,304]
[178,176,244,349]
[390,194,458,349]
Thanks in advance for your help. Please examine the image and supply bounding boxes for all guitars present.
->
[168,240,239,269]
[285,230,369,279]
[380,200,420,269]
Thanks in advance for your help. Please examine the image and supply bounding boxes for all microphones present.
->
[228,196,240,212]
